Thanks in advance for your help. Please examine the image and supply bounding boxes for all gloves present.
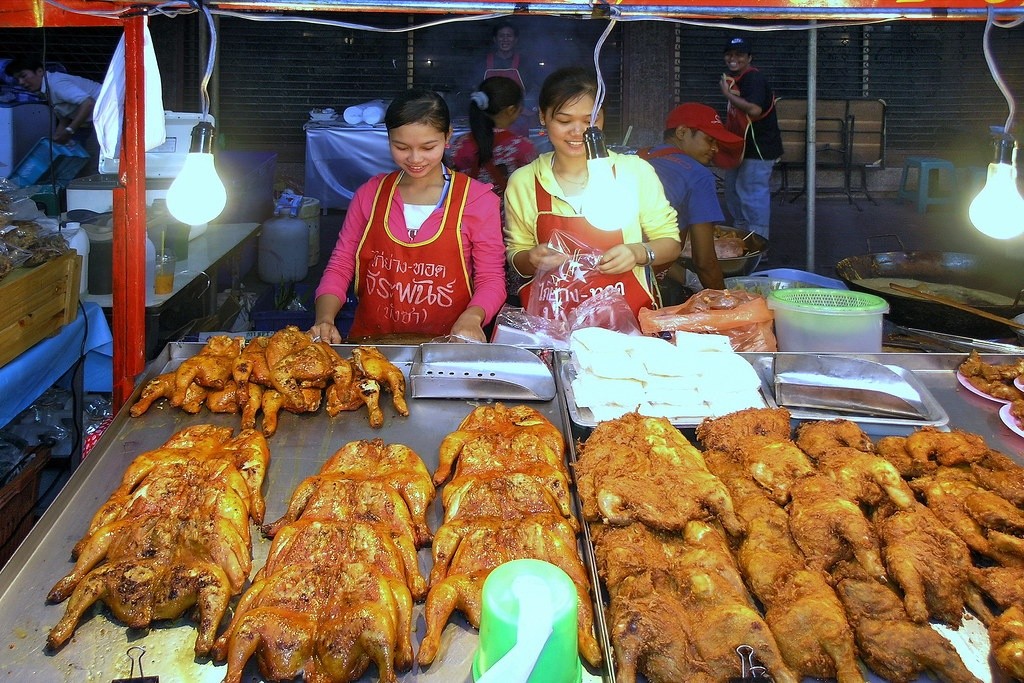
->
[683,269,704,294]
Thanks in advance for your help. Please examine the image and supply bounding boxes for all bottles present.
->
[258,207,309,283]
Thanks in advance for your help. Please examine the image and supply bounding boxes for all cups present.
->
[154,247,177,295]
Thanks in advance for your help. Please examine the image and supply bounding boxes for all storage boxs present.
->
[7,136,91,192]
[208,149,278,287]
[252,283,357,339]
[66,111,215,213]
[750,268,849,290]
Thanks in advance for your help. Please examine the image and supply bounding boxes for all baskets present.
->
[772,287,886,311]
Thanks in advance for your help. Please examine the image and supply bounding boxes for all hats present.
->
[664,103,743,149]
[723,35,752,56]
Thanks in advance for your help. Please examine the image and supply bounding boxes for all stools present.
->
[896,156,987,215]
[22,185,62,216]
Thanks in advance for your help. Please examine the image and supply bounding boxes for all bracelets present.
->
[65,127,74,135]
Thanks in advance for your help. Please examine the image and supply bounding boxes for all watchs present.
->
[637,243,655,267]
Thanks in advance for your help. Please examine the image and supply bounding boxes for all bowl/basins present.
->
[1009,319,1024,346]
[344,107,386,124]
[676,224,770,277]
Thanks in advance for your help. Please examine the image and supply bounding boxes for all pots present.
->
[837,234,1024,339]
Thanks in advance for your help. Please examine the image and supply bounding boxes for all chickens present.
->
[417,402,602,669]
[46,423,269,657]
[576,406,1024,683]
[130,325,409,441]
[210,437,437,683]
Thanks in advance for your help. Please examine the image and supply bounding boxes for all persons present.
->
[719,36,783,264]
[623,102,743,291]
[478,22,532,86]
[5,53,103,174]
[450,76,540,298]
[504,67,681,335]
[308,89,507,344]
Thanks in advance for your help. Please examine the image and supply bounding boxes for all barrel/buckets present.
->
[274,197,321,266]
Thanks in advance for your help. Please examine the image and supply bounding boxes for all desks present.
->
[88,223,263,359]
[0,302,113,475]
[303,113,552,217]
[775,95,886,210]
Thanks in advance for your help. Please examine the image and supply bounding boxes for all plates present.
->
[1014,377,1024,392]
[311,117,337,121]
[955,370,1015,404]
[999,403,1024,438]
